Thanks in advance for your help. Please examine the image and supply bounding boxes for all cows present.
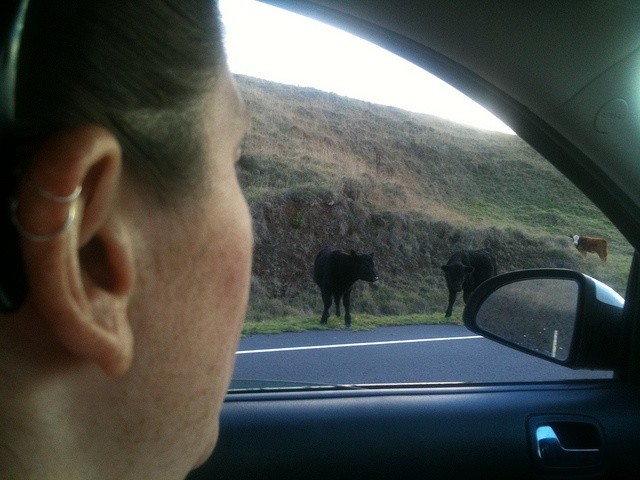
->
[569,234,608,263]
[313,248,380,331]
[441,247,499,318]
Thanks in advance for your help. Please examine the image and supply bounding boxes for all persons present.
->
[1,1,256,479]
